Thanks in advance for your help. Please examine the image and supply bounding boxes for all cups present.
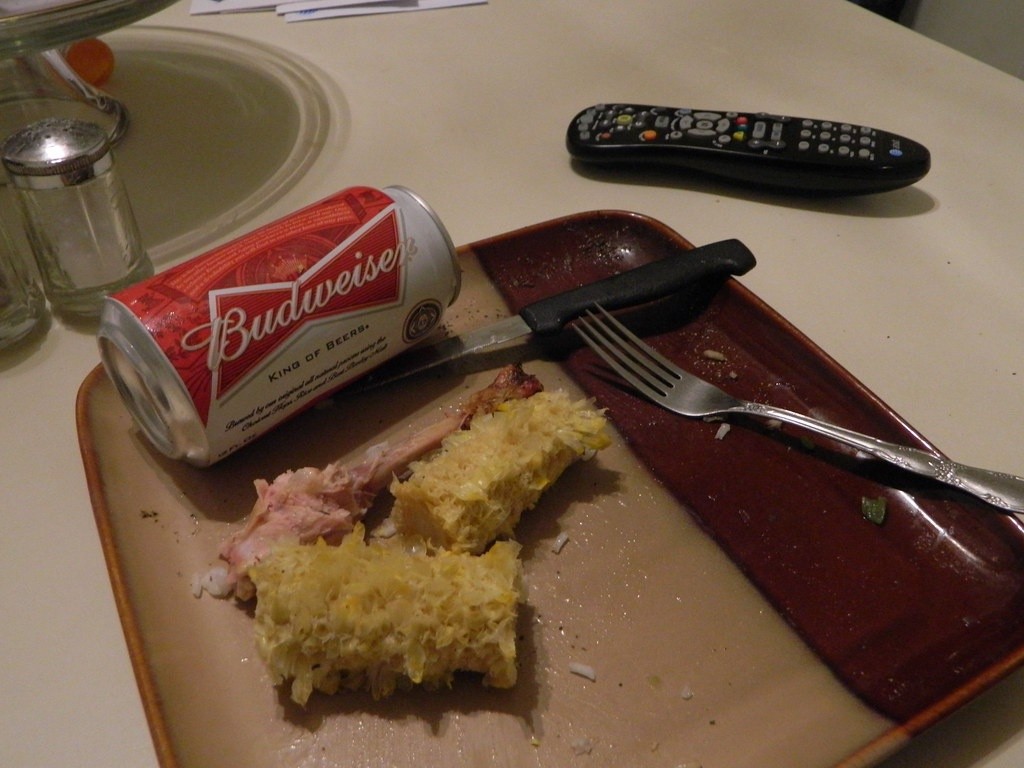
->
[0,113,158,346]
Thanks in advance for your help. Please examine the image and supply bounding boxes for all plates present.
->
[73,209,1024,768]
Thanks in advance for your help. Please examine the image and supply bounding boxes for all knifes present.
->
[340,237,756,383]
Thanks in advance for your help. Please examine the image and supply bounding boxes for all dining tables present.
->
[0,1,1023,768]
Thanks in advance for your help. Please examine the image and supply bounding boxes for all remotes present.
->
[566,102,931,196]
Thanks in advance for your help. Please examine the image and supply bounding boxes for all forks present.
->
[568,300,1022,523]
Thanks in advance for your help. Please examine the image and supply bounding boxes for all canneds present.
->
[98,185,462,467]
